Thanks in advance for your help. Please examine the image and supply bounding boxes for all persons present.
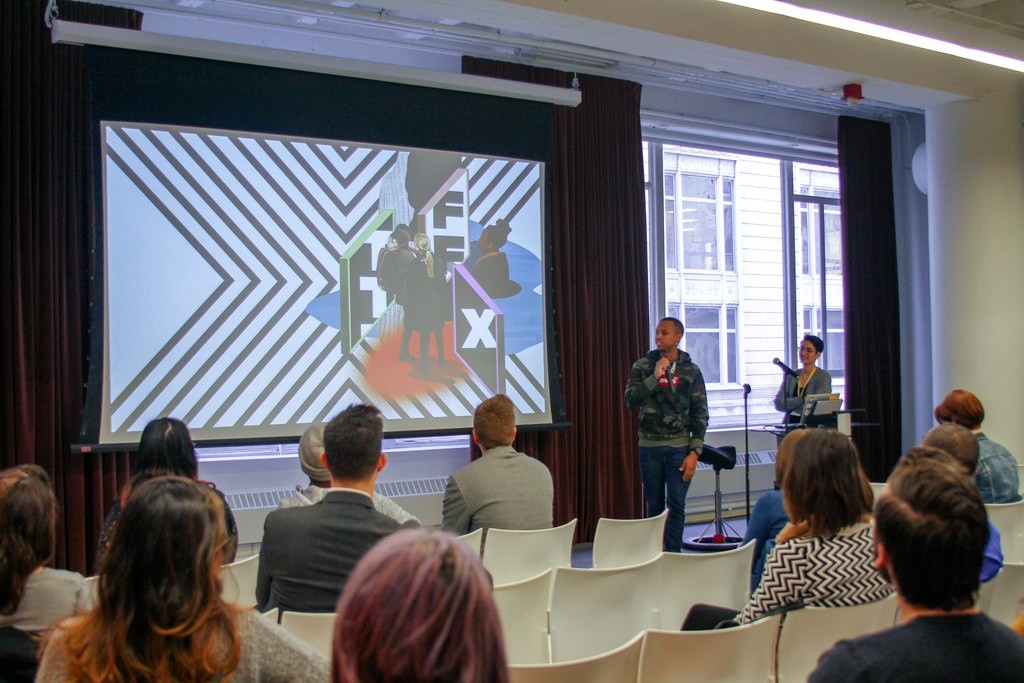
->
[921,424,1004,581]
[95,417,238,572]
[935,390,1019,504]
[279,424,421,526]
[809,460,1024,683]
[331,531,510,683]
[625,318,709,551]
[443,394,554,536]
[0,464,92,643]
[378,220,510,374]
[773,335,832,423]
[743,430,808,593]
[680,429,896,630]
[255,403,400,624]
[36,476,330,682]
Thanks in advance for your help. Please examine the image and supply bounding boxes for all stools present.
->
[683,443,744,547]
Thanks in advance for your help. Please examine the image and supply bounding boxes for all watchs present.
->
[690,447,702,456]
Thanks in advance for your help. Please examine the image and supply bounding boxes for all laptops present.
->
[773,393,843,429]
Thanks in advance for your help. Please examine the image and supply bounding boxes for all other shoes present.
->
[400,351,416,363]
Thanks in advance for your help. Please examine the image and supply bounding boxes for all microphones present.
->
[665,368,675,392]
[773,357,799,379]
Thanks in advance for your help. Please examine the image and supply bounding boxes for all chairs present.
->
[84,492,1024,683]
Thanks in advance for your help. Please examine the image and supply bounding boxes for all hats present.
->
[299,422,331,480]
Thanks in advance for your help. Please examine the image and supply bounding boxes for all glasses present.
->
[799,348,817,354]
